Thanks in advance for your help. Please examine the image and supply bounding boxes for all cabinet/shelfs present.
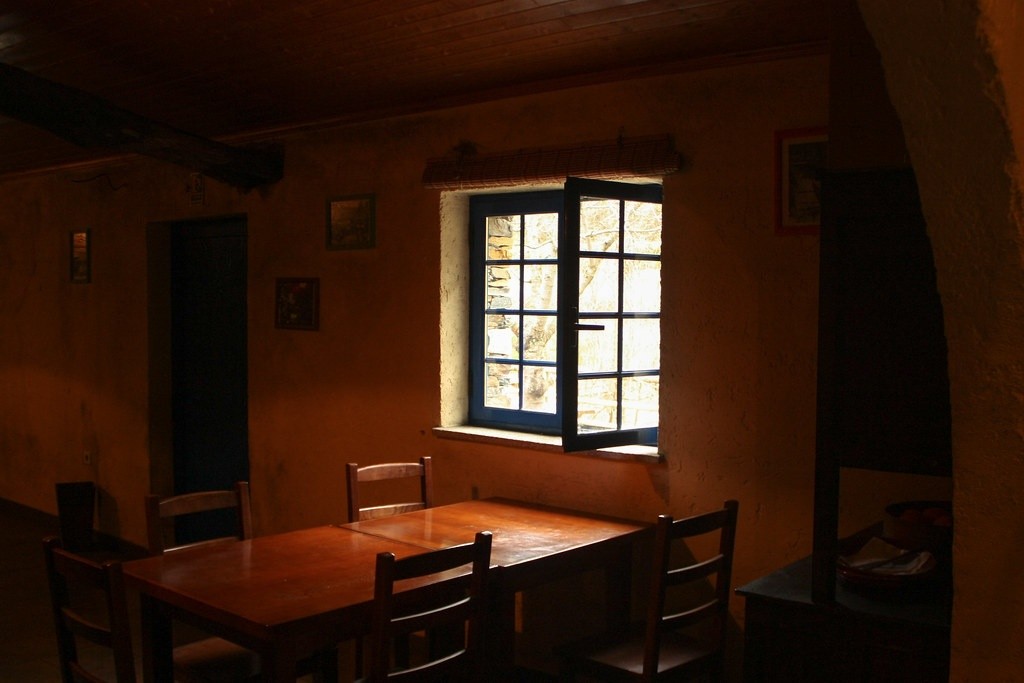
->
[732,521,952,683]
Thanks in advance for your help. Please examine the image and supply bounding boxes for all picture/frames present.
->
[67,228,91,285]
[274,277,320,331]
[772,126,829,237]
[324,192,375,251]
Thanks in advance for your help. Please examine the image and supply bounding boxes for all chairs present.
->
[347,455,465,678]
[145,480,339,683]
[346,531,492,683]
[42,534,135,683]
[551,499,739,683]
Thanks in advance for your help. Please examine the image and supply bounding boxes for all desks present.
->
[114,495,658,683]
